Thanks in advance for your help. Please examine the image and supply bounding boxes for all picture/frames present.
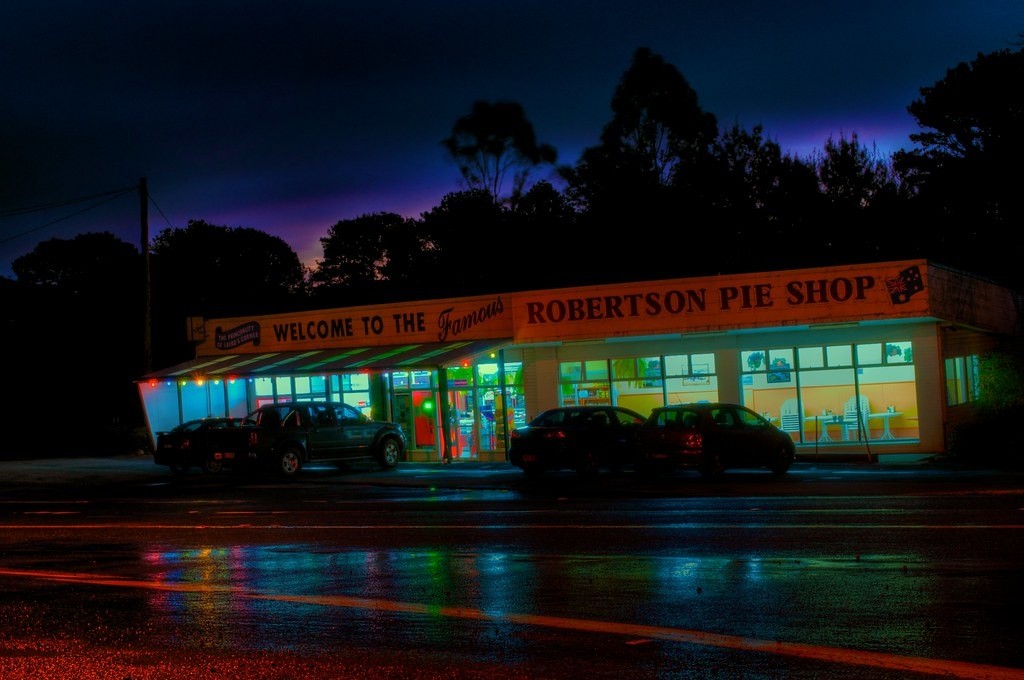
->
[682,364,710,386]
[639,368,663,388]
[766,363,790,383]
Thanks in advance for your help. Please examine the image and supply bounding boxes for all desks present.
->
[746,417,778,426]
[868,412,904,440]
[825,418,858,442]
[806,415,842,442]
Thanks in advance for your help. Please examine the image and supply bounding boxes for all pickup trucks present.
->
[206,402,408,478]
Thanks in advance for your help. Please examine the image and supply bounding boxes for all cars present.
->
[509,406,648,483]
[154,417,257,476]
[639,403,794,483]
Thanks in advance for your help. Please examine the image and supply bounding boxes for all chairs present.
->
[780,397,805,432]
[843,394,871,441]
